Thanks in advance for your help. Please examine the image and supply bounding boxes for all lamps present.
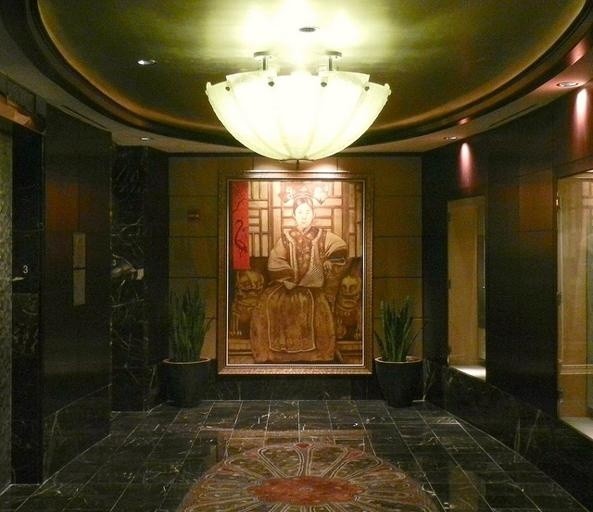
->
[200,25,393,167]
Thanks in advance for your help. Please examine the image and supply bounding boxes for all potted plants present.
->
[158,284,217,408]
[371,293,423,408]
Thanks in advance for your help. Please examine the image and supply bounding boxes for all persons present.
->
[249,198,347,362]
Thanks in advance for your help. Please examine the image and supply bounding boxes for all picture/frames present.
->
[215,169,373,374]
